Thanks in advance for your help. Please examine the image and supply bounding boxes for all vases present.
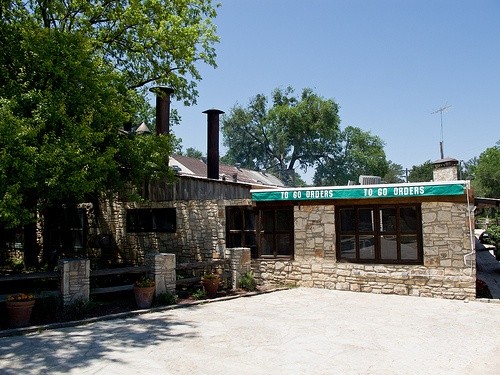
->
[7,301,35,328]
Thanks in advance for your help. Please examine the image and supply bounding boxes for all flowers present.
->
[7,294,33,302]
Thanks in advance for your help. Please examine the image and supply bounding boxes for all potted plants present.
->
[132,278,156,307]
[190,270,257,299]
